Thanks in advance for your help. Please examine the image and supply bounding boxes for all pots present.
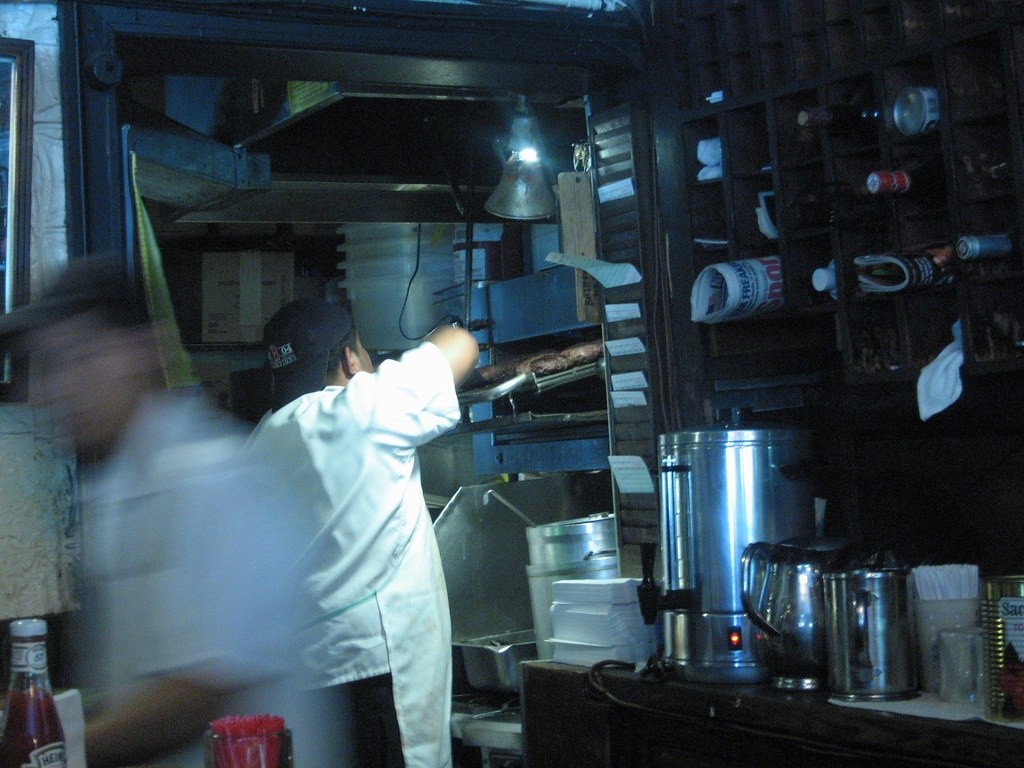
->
[524,513,619,660]
[635,404,817,682]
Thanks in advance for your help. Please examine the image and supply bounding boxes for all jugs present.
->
[822,563,919,702]
[739,529,865,693]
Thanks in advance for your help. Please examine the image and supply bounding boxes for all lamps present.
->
[482,94,558,222]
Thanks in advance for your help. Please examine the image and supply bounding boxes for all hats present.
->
[1,268,143,348]
[261,298,353,401]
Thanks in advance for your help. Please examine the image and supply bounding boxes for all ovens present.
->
[435,263,613,478]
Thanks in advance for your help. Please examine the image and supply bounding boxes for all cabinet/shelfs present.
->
[643,0,1024,413]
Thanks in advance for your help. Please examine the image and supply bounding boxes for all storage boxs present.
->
[203,248,298,347]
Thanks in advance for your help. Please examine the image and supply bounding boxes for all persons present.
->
[0,254,348,768]
[244,297,480,768]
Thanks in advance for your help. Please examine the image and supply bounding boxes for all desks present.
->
[517,656,1022,768]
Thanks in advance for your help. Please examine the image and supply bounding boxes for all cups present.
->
[203,730,294,768]
[914,598,979,692]
[940,627,988,705]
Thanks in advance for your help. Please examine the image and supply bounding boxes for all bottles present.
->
[796,92,880,140]
[0,619,68,768]
[867,157,948,209]
[956,229,1024,271]
[812,259,838,301]
